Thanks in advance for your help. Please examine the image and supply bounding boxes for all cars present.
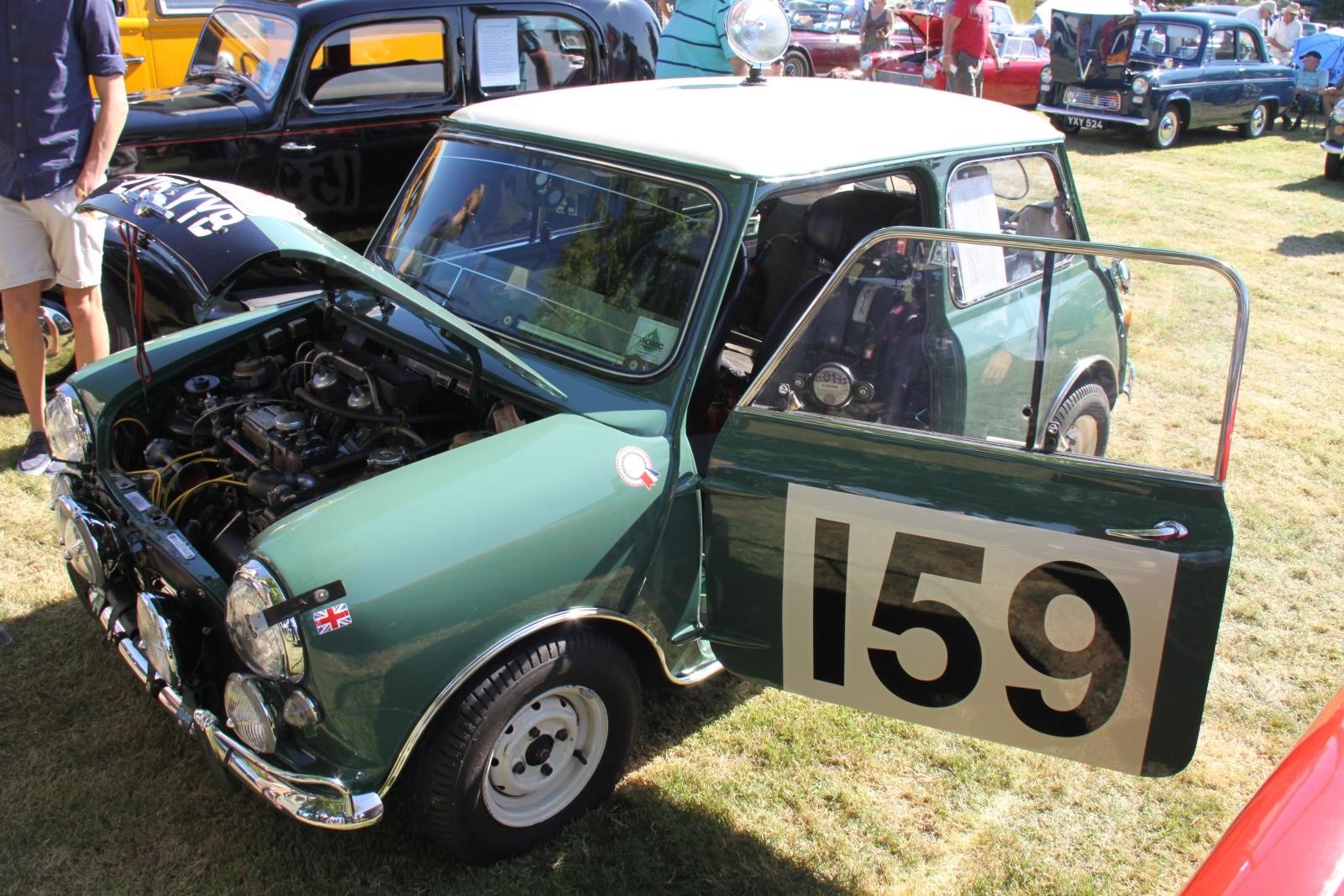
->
[860,9,1054,105]
[1320,99,1344,181]
[1033,6,1329,149]
[779,1,1045,76]
[0,0,665,411]
[42,68,1254,861]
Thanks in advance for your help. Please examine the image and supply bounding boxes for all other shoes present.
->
[1282,121,1292,129]
[1290,124,1300,132]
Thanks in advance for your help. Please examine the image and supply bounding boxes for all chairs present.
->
[1291,90,1321,129]
[743,189,932,432]
[601,217,750,385]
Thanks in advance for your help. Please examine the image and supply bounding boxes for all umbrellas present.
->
[1291,27,1344,85]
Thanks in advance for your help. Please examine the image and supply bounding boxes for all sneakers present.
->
[16,431,53,476]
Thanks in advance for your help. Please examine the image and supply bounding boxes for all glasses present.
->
[459,214,468,236]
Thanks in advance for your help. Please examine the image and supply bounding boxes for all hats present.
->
[1301,9,1306,15]
[1299,51,1322,60]
[1260,1,1280,15]
[1281,1,1301,15]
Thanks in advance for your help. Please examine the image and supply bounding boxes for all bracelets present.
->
[1101,58,1104,60]
[1305,88,1309,93]
[993,54,998,58]
[944,55,950,57]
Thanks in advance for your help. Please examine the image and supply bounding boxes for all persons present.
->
[848,0,895,57]
[1034,29,1050,56]
[467,5,552,101]
[1094,15,1118,77]
[1267,2,1303,64]
[1075,15,1092,73]
[1236,0,1277,41]
[942,0,1003,98]
[1281,51,1329,133]
[1295,9,1309,23]
[970,298,1044,438]
[1318,77,1344,130]
[0,0,129,479]
[395,184,485,289]
[1130,0,1157,12]
[655,0,784,80]
